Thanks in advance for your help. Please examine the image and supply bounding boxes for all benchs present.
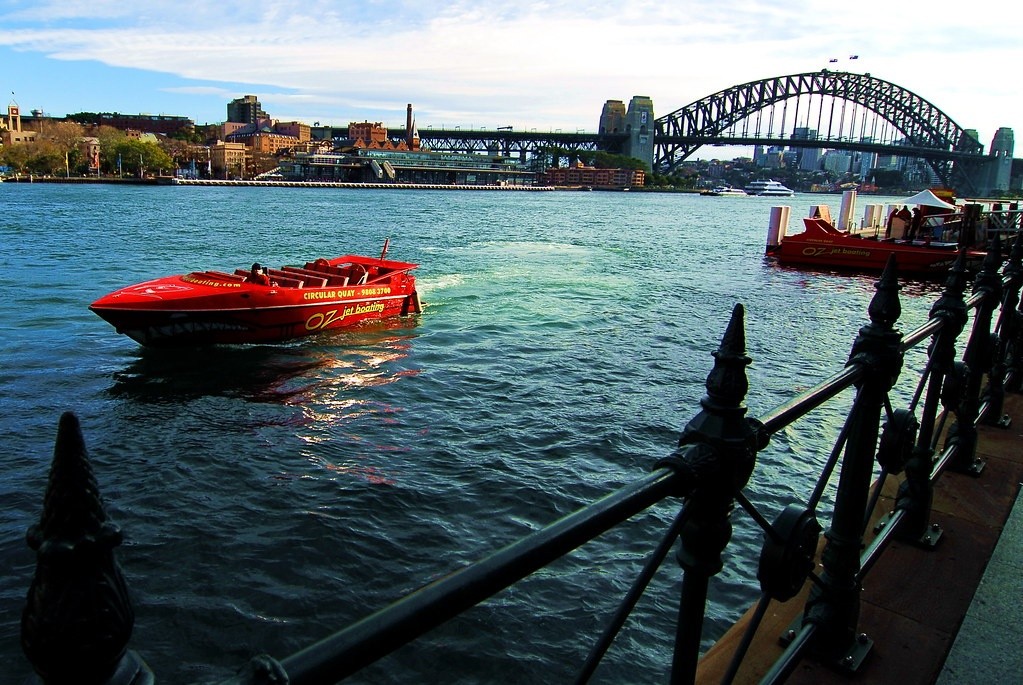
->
[205,262,382,290]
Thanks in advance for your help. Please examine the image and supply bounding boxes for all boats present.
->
[803,181,861,194]
[700,186,748,196]
[88,239,431,351]
[780,217,1009,278]
[743,178,794,197]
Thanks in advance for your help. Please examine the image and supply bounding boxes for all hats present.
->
[252,262,260,271]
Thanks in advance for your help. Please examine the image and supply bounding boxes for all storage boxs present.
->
[926,217,945,226]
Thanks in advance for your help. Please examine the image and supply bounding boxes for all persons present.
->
[908,208,921,241]
[246,262,270,286]
[897,204,911,221]
[884,208,899,239]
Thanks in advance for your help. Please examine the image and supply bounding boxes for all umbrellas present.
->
[901,189,957,217]
